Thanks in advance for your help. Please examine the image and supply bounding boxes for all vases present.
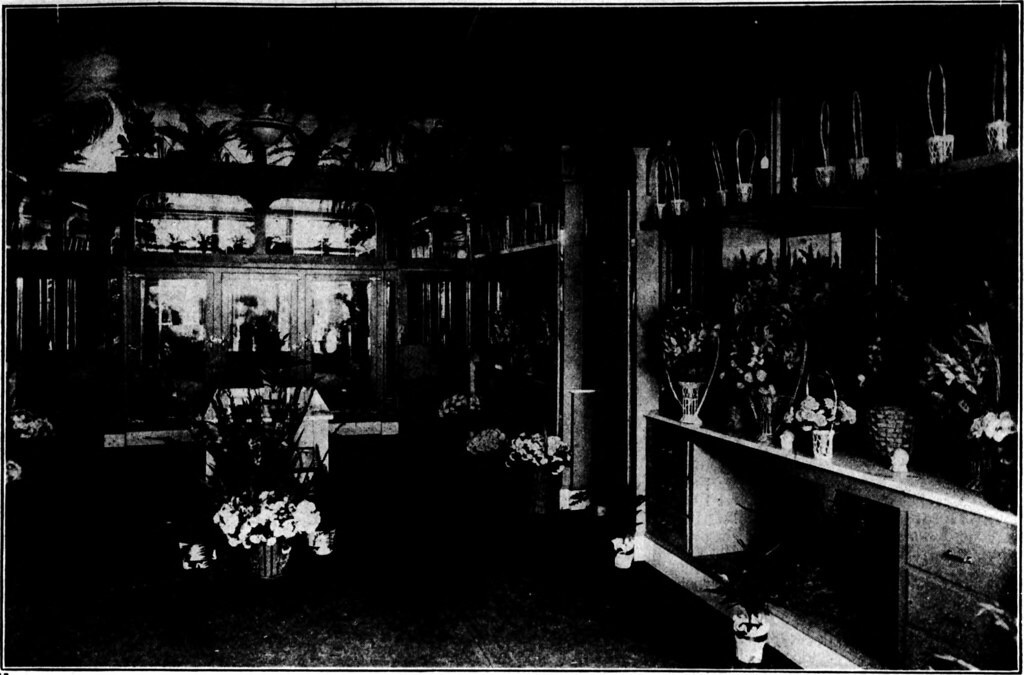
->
[980,436,1016,510]
[814,430,832,460]
[614,553,634,571]
[732,634,767,663]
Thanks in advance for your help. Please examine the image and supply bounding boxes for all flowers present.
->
[730,606,769,638]
[912,311,1013,444]
[609,537,636,552]
[787,394,858,431]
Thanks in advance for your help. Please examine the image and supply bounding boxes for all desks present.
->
[640,413,1018,671]
